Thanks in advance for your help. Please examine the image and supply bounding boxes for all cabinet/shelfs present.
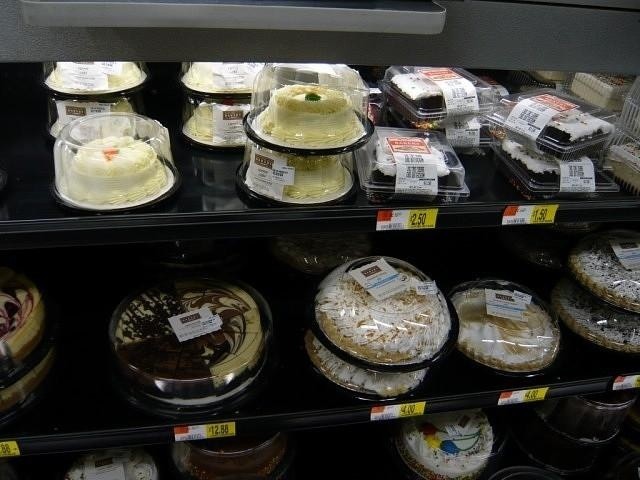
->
[0,0,640,480]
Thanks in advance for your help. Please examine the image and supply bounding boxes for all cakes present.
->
[0,265,59,413]
[45,62,640,210]
[65,221,640,480]
